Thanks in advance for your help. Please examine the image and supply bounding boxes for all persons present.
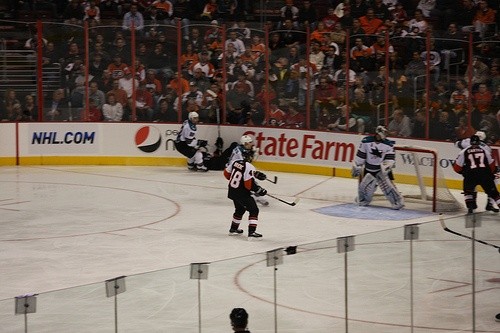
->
[230,308,251,333]
[225,135,270,207]
[352,126,405,210]
[224,149,267,240]
[0,0,500,146]
[456,131,500,213]
[174,112,259,172]
[453,135,500,209]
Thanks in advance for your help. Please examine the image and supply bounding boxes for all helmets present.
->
[230,309,248,330]
[470,135,481,145]
[240,135,252,146]
[242,149,255,161]
[375,126,388,139]
[475,131,486,141]
[188,111,199,120]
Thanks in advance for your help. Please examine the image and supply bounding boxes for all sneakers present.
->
[485,199,500,214]
[248,232,263,241]
[467,209,473,213]
[196,165,208,172]
[228,228,243,236]
[187,166,197,170]
[257,198,270,207]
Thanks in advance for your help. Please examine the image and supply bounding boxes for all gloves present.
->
[254,171,266,181]
[197,139,207,146]
[254,186,267,196]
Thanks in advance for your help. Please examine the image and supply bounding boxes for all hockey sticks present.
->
[438,213,500,249]
[266,176,278,184]
[215,108,222,156]
[266,193,300,206]
